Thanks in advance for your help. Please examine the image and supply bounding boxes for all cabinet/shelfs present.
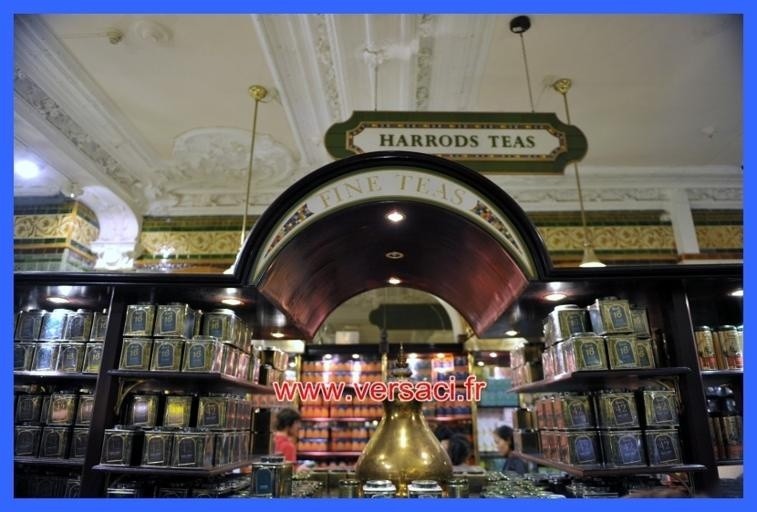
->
[268,336,527,473]
[13,271,312,498]
[507,262,743,499]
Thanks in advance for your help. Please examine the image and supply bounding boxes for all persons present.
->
[493,425,525,477]
[273,406,311,475]
[434,426,453,451]
[448,434,473,466]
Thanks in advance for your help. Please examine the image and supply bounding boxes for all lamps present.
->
[549,77,611,269]
